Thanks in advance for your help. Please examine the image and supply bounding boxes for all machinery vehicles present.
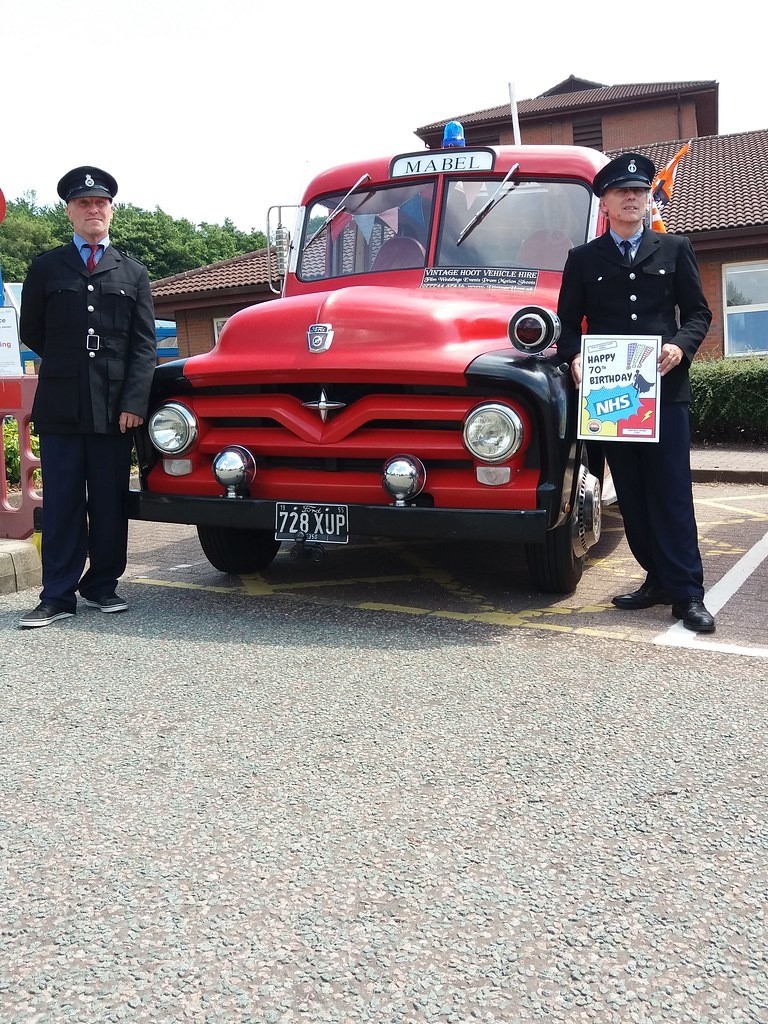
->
[127,121,617,596]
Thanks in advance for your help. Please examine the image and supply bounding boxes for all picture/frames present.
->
[576,334,662,443]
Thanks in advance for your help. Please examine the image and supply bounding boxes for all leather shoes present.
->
[612,578,674,610]
[672,595,715,630]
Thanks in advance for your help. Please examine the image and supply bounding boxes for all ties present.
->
[620,241,633,265]
[82,244,103,274]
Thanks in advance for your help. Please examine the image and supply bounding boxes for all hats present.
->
[592,153,655,198]
[57,166,118,206]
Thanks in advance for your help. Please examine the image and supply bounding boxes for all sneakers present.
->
[86,592,129,612]
[18,601,75,626]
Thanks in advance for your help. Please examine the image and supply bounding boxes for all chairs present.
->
[513,229,574,271]
[368,236,426,272]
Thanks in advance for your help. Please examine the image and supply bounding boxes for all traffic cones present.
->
[651,198,666,234]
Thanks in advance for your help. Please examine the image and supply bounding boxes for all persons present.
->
[556,152,716,632]
[15,164,157,629]
